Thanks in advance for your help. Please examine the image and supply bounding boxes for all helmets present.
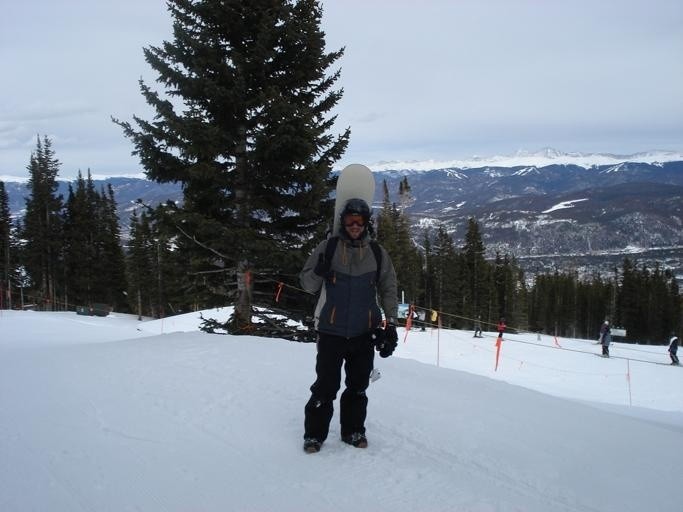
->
[341,198,370,223]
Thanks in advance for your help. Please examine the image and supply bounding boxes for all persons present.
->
[473,314,481,337]
[408,308,438,332]
[298,197,399,454]
[666,330,680,366]
[497,317,505,338]
[599,319,611,356]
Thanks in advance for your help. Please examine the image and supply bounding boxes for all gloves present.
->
[314,252,331,277]
[384,323,398,351]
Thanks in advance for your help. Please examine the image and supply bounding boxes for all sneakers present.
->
[304,437,324,452]
[341,431,367,448]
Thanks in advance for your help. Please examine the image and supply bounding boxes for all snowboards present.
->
[314,164,375,332]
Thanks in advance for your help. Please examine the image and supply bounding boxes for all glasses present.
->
[341,211,370,226]
